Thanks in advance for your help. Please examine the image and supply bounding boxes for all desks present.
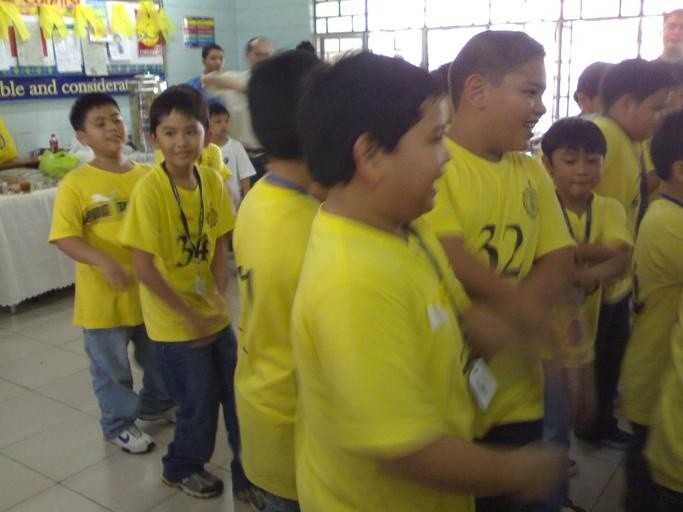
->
[0,166,75,314]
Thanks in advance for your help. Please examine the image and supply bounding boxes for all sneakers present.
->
[163,467,222,500]
[140,404,181,421]
[577,417,633,452]
[110,425,155,455]
[550,449,579,476]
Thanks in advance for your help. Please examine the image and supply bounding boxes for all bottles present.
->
[50,132,58,153]
[0,173,20,196]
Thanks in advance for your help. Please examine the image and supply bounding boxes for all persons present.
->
[647,9,683,65]
[229,52,326,509]
[536,57,683,511]
[423,29,576,509]
[155,37,276,212]
[47,91,180,455]
[293,49,572,509]
[118,83,241,500]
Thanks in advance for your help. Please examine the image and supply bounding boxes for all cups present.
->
[16,171,32,194]
